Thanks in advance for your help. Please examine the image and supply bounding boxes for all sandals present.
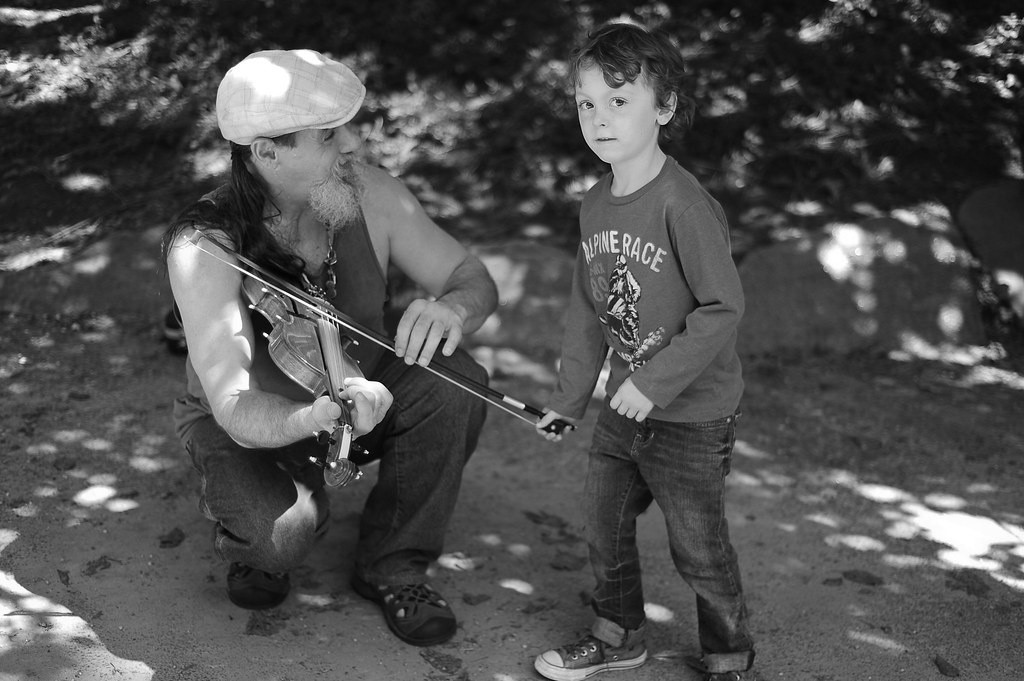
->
[348,558,456,645]
[213,522,291,610]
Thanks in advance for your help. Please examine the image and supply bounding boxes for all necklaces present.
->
[298,220,337,298]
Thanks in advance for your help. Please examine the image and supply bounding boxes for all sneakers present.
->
[534,634,647,681]
[707,670,743,681]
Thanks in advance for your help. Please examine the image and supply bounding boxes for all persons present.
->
[159,47,500,644]
[536,23,757,681]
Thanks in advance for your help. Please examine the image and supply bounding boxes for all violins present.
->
[242,261,367,489]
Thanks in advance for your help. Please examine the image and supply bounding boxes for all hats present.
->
[217,48,368,146]
